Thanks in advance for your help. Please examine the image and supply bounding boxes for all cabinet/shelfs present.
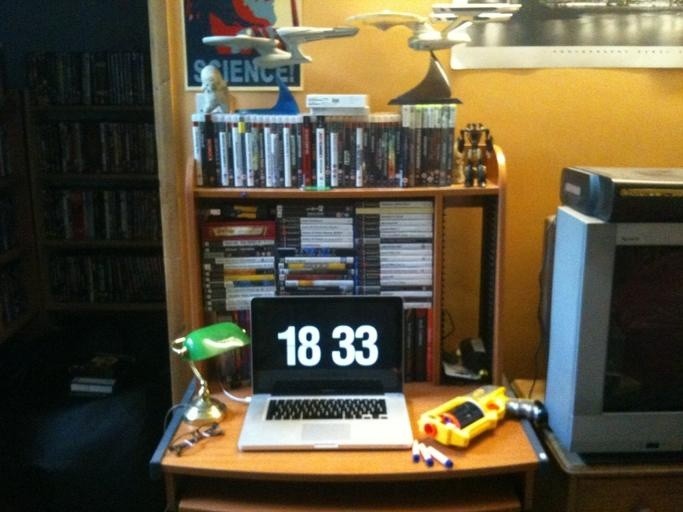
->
[190,143,506,386]
[25,51,166,313]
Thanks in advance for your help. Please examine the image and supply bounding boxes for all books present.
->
[206,310,250,383]
[405,309,434,382]
[72,383,115,395]
[200,200,434,314]
[26,50,166,305]
[191,104,455,190]
[72,349,129,383]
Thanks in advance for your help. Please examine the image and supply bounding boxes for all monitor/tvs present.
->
[537,207,683,469]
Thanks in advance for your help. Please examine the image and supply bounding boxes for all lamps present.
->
[169,321,250,425]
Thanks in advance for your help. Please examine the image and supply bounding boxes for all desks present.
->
[146,379,550,512]
[513,377,683,512]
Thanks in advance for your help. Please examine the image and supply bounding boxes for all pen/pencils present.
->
[412,439,453,467]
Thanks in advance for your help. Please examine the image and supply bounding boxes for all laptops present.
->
[237,294,415,452]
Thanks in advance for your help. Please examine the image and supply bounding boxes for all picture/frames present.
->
[181,0,304,91]
